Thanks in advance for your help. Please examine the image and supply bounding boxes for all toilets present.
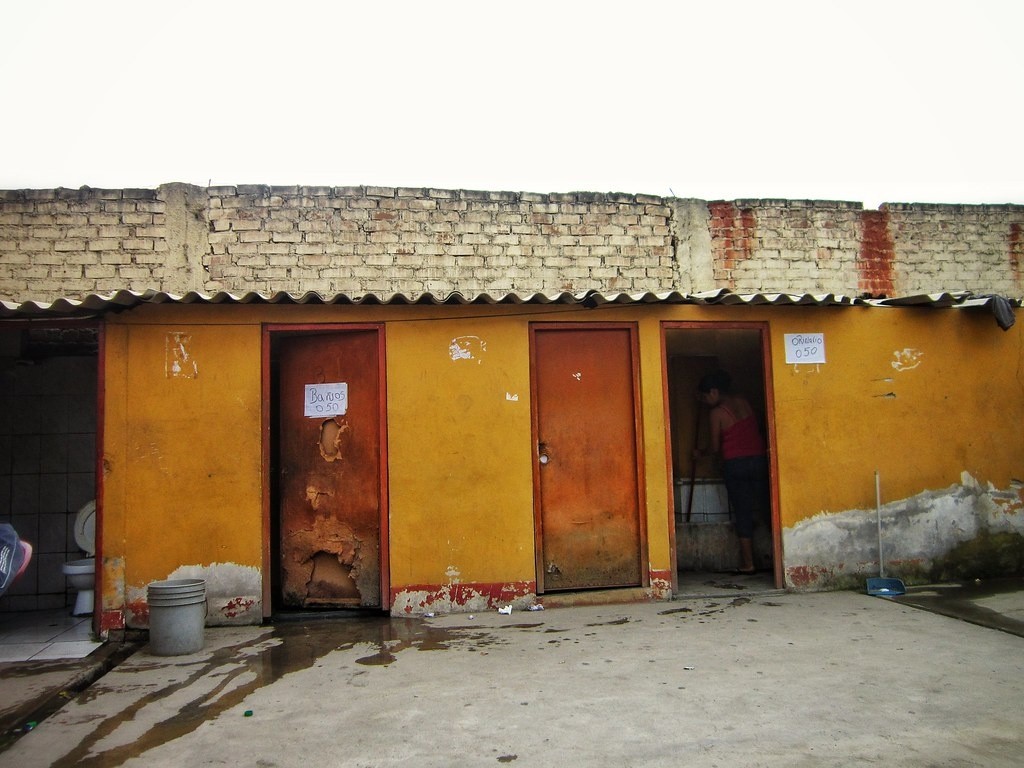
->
[62,500,96,616]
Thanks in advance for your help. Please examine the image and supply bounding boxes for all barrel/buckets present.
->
[146,578,208,658]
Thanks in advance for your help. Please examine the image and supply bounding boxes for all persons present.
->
[688,370,772,575]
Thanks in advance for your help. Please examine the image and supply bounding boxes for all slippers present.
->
[733,565,757,575]
